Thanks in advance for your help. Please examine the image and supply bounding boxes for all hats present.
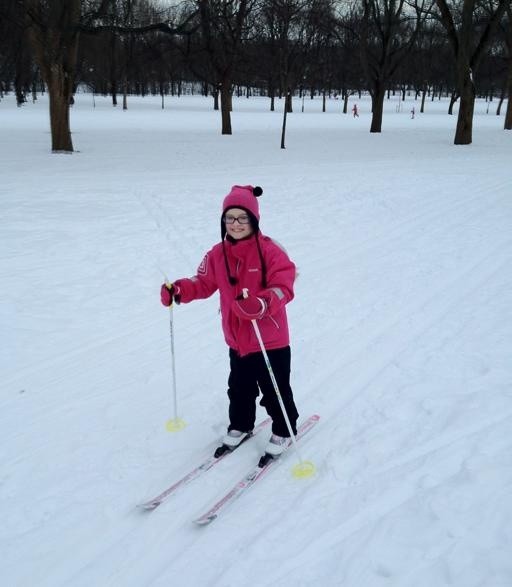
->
[221,185,267,288]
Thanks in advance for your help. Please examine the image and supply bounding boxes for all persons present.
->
[161,185,299,456]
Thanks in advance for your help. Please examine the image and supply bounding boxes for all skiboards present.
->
[136,413,320,525]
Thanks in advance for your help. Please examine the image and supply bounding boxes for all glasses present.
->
[221,217,250,225]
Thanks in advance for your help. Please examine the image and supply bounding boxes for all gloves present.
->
[160,282,180,306]
[231,294,267,320]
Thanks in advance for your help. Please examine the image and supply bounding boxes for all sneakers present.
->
[264,434,291,455]
[223,431,248,446]
